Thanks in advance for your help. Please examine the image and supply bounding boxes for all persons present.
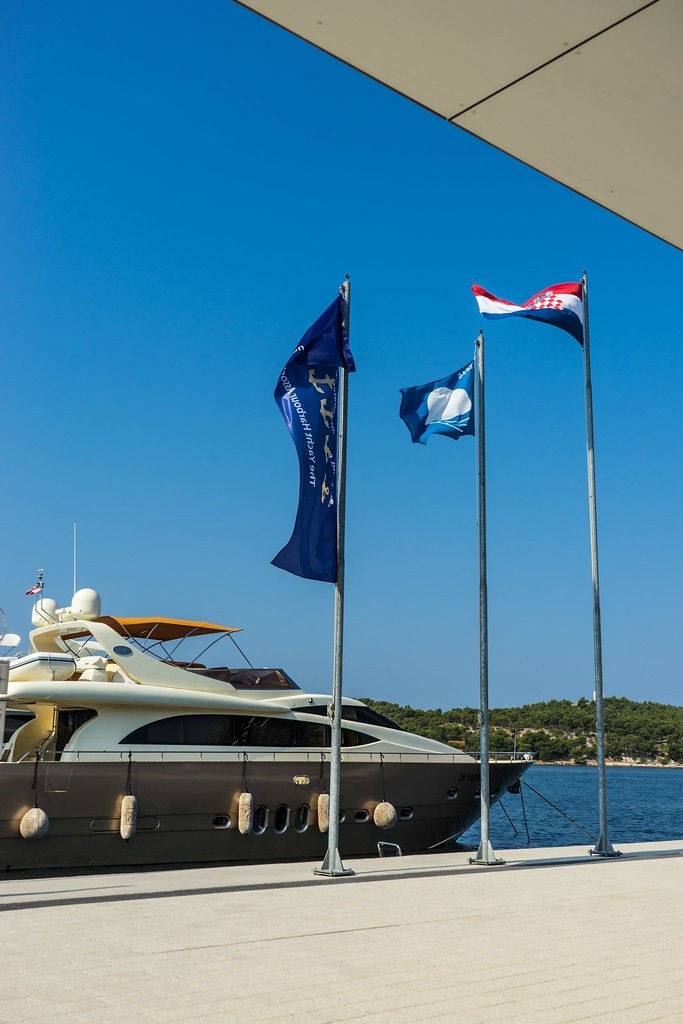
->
[268,295,357,583]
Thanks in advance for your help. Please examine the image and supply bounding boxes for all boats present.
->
[0,517,539,881]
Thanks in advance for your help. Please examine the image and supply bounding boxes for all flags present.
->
[471,282,586,350]
[26,586,42,596]
[399,362,476,445]
